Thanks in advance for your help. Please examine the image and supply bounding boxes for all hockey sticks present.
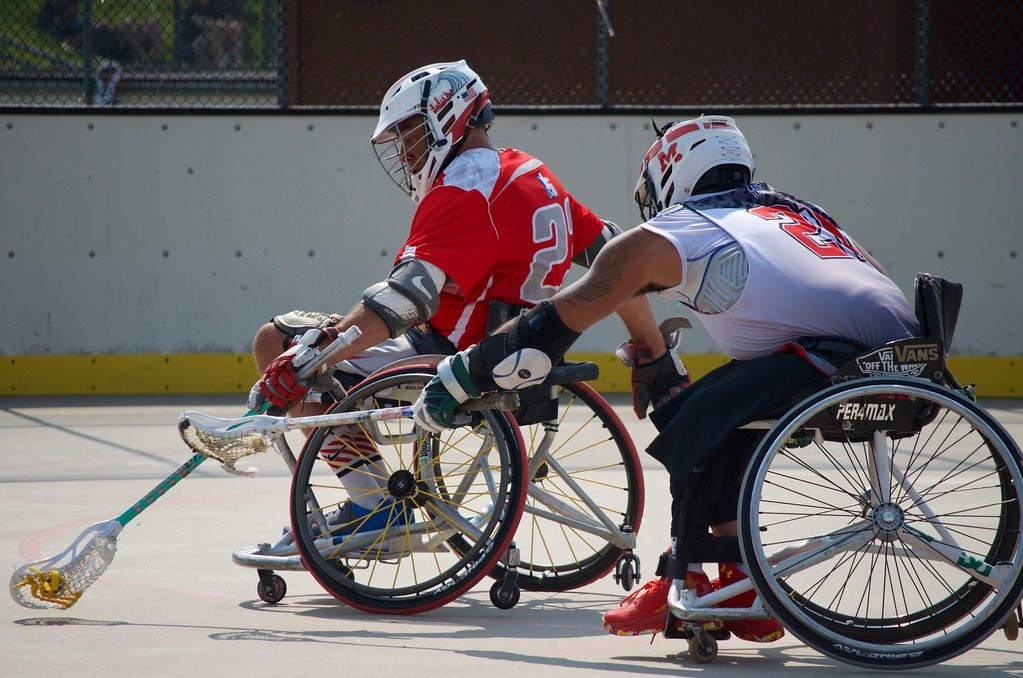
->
[180,390,518,478]
[8,323,366,610]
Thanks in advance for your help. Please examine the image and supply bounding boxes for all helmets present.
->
[369,58,495,206]
[632,113,756,222]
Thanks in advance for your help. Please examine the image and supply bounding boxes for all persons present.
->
[414,113,922,646]
[253,60,691,551]
[62,17,169,70]
[183,0,249,68]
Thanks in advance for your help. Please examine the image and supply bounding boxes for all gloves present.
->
[412,344,500,434]
[630,330,693,420]
[245,328,327,409]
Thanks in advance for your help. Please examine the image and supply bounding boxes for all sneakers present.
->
[705,562,785,642]
[600,566,725,636]
[282,496,415,553]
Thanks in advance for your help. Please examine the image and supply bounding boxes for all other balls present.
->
[41,573,66,595]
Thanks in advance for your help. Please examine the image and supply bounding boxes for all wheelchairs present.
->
[663,274,1023,673]
[226,350,647,615]
